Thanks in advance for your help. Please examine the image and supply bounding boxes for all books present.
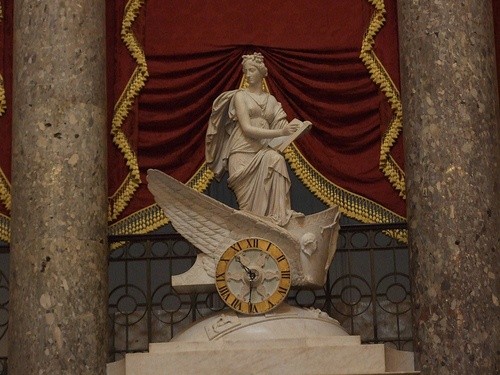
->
[267,115,313,155]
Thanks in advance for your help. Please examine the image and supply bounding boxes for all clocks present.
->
[215,237,292,316]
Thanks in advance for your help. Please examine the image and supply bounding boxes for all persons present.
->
[220,51,306,221]
[299,232,318,258]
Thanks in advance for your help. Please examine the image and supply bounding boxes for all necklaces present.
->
[246,90,271,116]
